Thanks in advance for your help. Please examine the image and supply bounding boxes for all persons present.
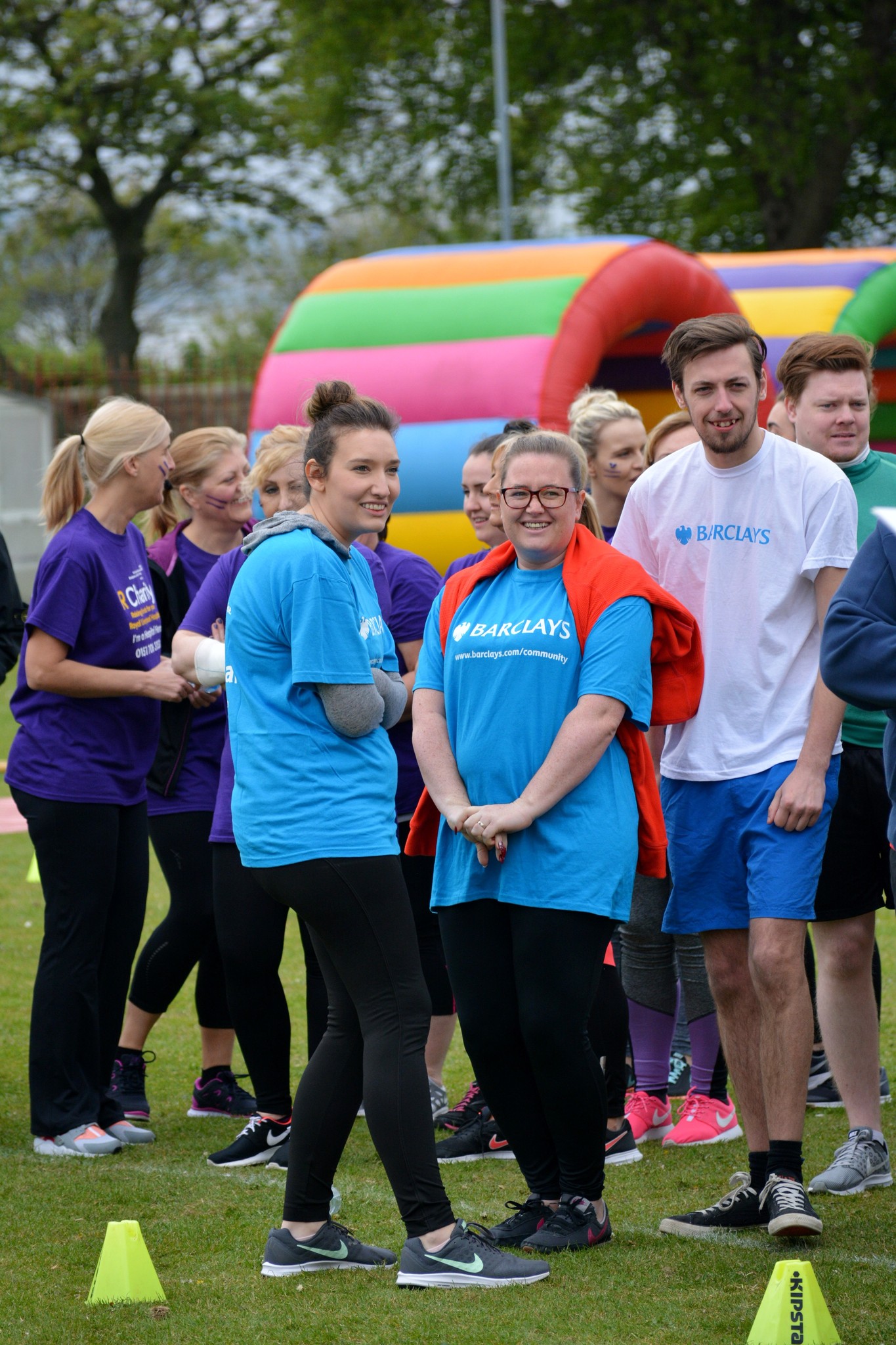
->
[412,433,704,1253]
[227,378,549,1288]
[427,392,896,1195]
[173,425,391,1165]
[0,533,28,683]
[608,314,856,1240]
[113,425,260,1130]
[356,510,457,1123]
[5,397,221,1155]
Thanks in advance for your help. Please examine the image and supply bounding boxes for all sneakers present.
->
[104,1120,156,1145]
[433,1080,485,1131]
[662,1086,743,1147]
[604,1118,643,1166]
[758,1169,823,1237]
[478,1193,555,1249]
[624,1064,635,1093]
[623,1091,674,1145]
[260,1214,397,1278]
[435,1106,516,1164]
[206,1107,293,1167]
[666,1051,692,1099]
[187,1071,257,1120]
[807,1127,894,1197]
[806,1067,892,1108]
[395,1218,550,1291]
[105,1050,156,1121]
[428,1077,447,1121]
[33,1122,122,1158]
[265,1140,290,1171]
[808,1050,831,1091]
[659,1171,770,1240]
[520,1193,612,1255]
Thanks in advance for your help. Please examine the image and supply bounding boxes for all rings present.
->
[477,821,486,829]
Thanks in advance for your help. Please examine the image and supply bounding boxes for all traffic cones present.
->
[82,1220,166,1305]
[25,850,40,882]
[747,1260,840,1345]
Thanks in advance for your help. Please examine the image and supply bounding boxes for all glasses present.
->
[501,487,576,509]
